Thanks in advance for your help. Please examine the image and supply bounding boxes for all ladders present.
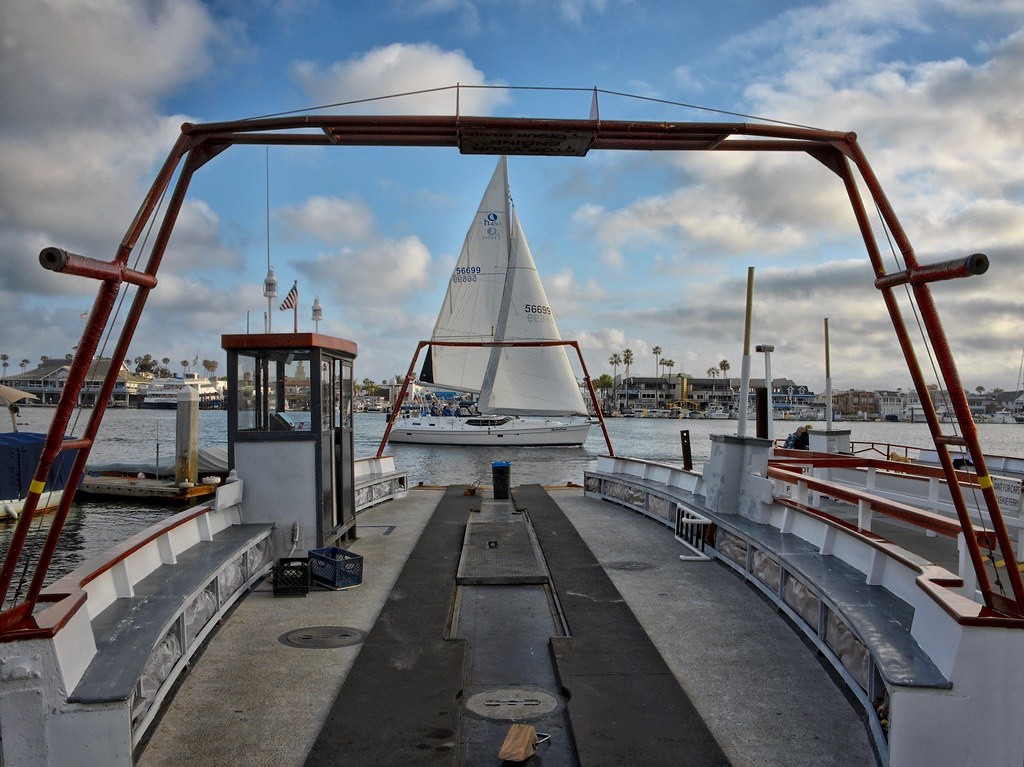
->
[674,502,712,562]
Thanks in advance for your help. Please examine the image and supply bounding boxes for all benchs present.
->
[583,440,1024,767]
[0,499,277,767]
[354,455,409,512]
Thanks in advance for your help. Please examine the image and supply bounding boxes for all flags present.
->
[80,313,86,319]
[279,285,298,312]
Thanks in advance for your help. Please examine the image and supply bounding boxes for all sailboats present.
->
[386,154,603,448]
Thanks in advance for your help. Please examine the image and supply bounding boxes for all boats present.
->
[0,384,80,524]
[587,390,1024,425]
[139,368,213,414]
[75,415,221,507]
[246,372,480,418]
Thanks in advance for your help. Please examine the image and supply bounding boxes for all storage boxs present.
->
[272,557,311,598]
[308,546,364,591]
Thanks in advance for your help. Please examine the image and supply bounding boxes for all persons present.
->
[454,408,461,418]
[431,402,439,417]
[442,403,452,416]
